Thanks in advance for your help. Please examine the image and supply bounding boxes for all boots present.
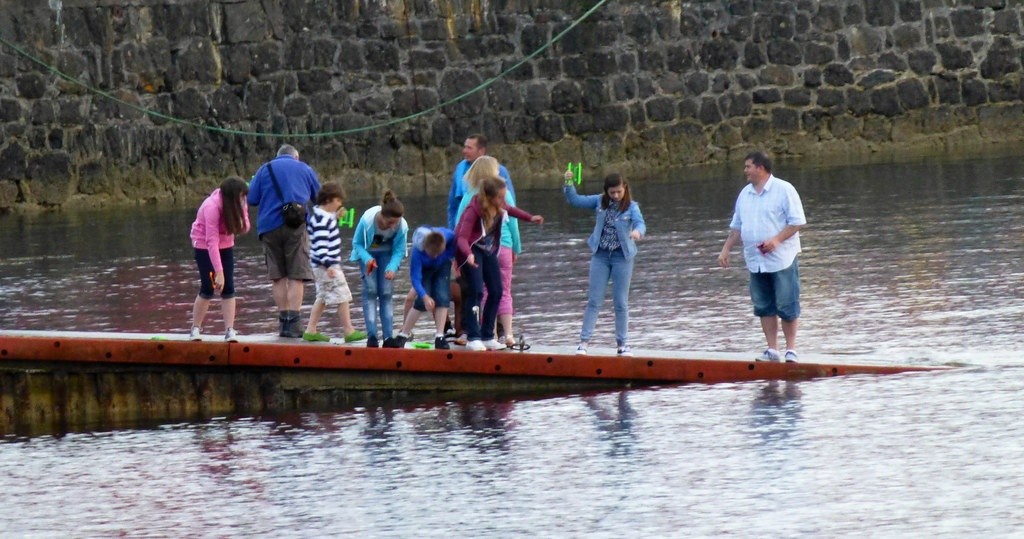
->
[287,310,306,338]
[279,310,290,337]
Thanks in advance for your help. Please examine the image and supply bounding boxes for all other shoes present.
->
[190,326,202,341]
[393,335,409,347]
[444,328,457,342]
[382,338,396,348]
[455,333,468,345]
[343,330,367,342]
[435,337,450,349]
[225,328,238,343]
[367,336,379,347]
[505,338,518,349]
[303,331,331,341]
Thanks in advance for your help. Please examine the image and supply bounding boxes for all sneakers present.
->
[483,339,507,350]
[754,350,781,362]
[617,348,633,356]
[576,344,588,356]
[466,339,487,352]
[784,351,798,362]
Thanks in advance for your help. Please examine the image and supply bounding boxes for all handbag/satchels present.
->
[281,202,307,228]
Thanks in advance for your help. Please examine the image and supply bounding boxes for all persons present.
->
[447,132,516,344]
[247,144,321,338]
[455,154,521,347]
[350,186,409,347]
[453,175,544,352]
[394,225,456,349]
[190,176,250,342]
[564,169,645,356]
[302,179,367,342]
[718,150,807,363]
